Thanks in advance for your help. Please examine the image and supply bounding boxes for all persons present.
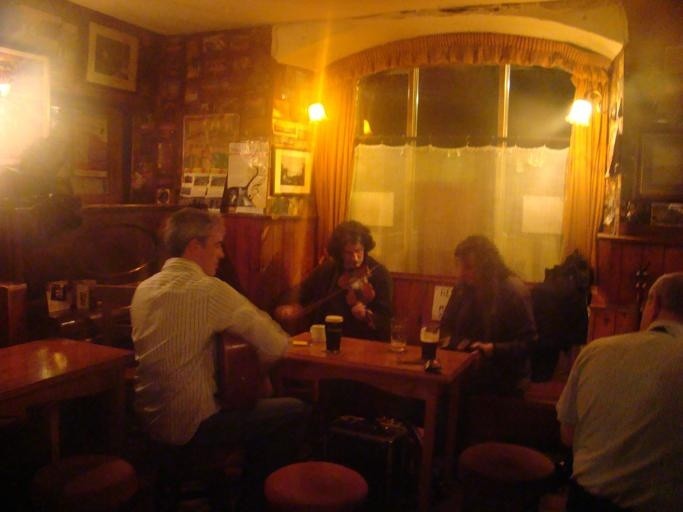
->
[554,272,682,511]
[129,205,311,512]
[437,234,538,397]
[522,247,592,383]
[298,220,394,346]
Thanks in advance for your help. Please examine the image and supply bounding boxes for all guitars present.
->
[216,330,256,409]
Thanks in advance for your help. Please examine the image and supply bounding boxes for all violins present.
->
[338,264,375,322]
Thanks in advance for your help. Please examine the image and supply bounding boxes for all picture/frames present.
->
[628,123,683,203]
[83,18,141,97]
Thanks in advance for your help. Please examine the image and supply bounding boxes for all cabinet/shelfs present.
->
[587,228,683,349]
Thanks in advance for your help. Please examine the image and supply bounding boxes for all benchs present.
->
[384,271,577,406]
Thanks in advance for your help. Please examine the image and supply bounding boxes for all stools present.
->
[31,452,140,510]
[459,439,556,511]
[265,458,368,511]
[146,447,234,510]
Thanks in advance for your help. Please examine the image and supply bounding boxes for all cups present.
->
[418,326,441,361]
[391,315,410,354]
[310,344,326,358]
[324,314,345,353]
[311,325,327,343]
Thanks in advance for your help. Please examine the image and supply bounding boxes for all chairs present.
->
[94,281,138,439]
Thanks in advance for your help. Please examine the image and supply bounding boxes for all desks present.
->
[2,334,138,459]
[275,329,473,511]
